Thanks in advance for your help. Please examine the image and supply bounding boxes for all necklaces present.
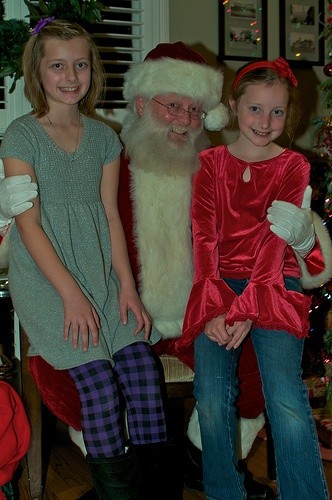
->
[45,113,80,158]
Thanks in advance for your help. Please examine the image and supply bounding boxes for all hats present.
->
[121,42,229,131]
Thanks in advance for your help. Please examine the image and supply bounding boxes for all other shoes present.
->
[185,449,276,499]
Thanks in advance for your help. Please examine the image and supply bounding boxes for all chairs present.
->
[18,314,277,500]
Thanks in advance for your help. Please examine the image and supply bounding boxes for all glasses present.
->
[152,98,207,120]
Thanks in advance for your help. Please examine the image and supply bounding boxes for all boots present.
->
[85,455,132,500]
[132,441,182,499]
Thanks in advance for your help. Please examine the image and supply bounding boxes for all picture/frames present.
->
[217,0,268,62]
[279,0,325,67]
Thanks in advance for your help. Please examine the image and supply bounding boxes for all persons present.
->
[0,15,182,500]
[174,56,329,500]
[0,42,332,500]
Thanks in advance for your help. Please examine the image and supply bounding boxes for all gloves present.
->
[0,158,38,228]
[266,185,316,258]
